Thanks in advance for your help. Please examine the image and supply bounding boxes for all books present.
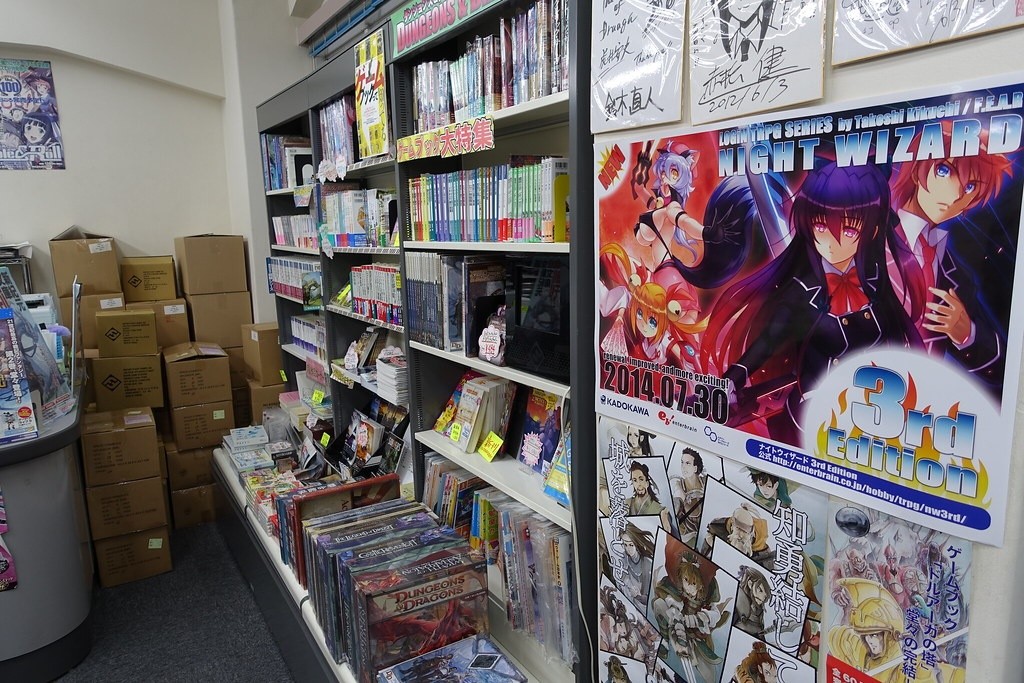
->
[278,371,333,431]
[340,392,408,476]
[272,215,318,250]
[421,451,573,670]
[320,95,356,164]
[376,355,409,406]
[291,314,326,360]
[220,424,327,537]
[351,327,389,368]
[329,263,403,326]
[516,387,574,512]
[265,256,321,301]
[405,252,506,361]
[411,0,570,135]
[260,133,314,192]
[409,158,570,243]
[320,182,400,248]
[433,370,514,454]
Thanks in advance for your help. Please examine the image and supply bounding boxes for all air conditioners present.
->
[296,0,407,60]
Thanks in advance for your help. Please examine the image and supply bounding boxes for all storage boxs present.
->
[58,293,125,354]
[161,341,233,408]
[0,266,76,426]
[241,320,288,387]
[80,405,159,488]
[184,291,252,350]
[75,349,165,412]
[269,472,529,683]
[94,525,173,586]
[242,376,289,427]
[154,432,225,530]
[95,309,159,358]
[125,297,190,350]
[0,307,38,445]
[47,224,122,298]
[119,254,178,302]
[173,232,248,293]
[84,475,167,539]
[169,399,235,453]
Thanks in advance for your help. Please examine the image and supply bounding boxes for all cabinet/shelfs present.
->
[210,447,540,683]
[254,18,420,501]
[386,0,593,683]
